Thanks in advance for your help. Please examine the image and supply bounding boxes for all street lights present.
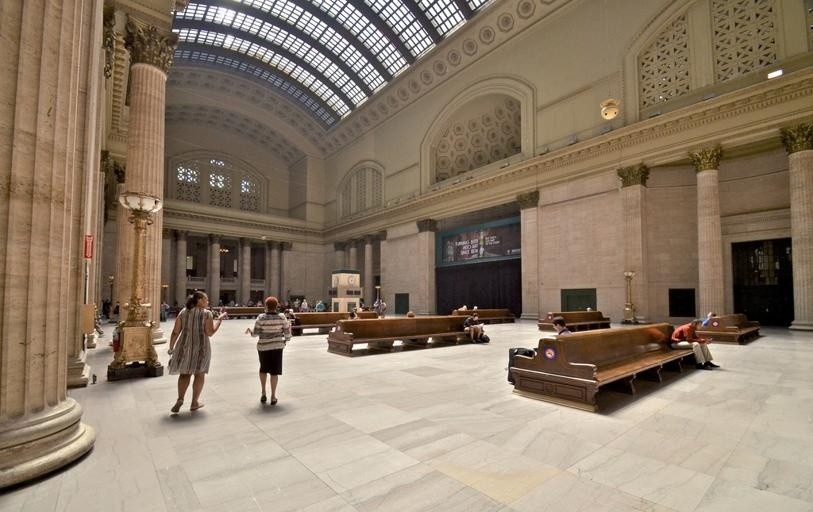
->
[161,282,168,303]
[621,269,634,304]
[374,286,381,300]
[117,190,163,326]
[106,274,115,305]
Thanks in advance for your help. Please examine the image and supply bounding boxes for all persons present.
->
[669,318,720,370]
[101,298,119,322]
[214,297,327,313]
[462,312,482,343]
[551,316,572,337]
[166,290,228,413]
[347,298,387,320]
[701,310,717,328]
[243,296,291,406]
[641,328,669,352]
[160,300,171,322]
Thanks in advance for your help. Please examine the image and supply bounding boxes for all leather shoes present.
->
[260,396,279,406]
[190,402,205,410]
[170,399,184,413]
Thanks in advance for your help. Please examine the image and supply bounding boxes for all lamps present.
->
[106,189,164,380]
[599,79,621,120]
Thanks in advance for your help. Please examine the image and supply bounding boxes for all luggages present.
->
[507,347,535,382]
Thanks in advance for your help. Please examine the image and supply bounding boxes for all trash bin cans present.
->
[290,319,302,336]
[159,304,166,322]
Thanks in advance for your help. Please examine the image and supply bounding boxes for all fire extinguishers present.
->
[112,327,120,352]
